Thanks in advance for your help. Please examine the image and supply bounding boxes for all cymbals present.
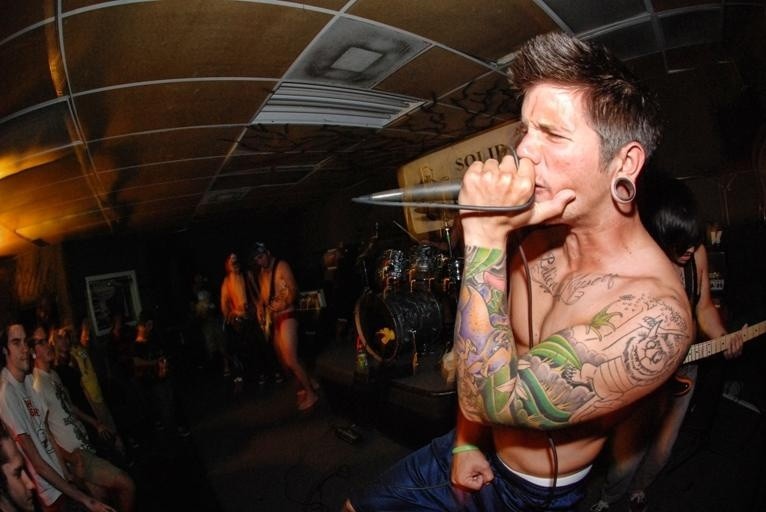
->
[415,204,458,214]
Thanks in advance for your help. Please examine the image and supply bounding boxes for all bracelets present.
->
[450,444,480,455]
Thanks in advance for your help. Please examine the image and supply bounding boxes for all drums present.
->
[442,256,464,291]
[408,244,438,280]
[377,249,408,281]
[354,288,443,364]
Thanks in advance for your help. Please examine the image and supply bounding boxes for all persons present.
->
[217,252,267,398]
[589,170,748,512]
[0,314,174,509]
[338,29,694,512]
[248,239,321,410]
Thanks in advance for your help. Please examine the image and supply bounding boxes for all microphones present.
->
[357,177,463,202]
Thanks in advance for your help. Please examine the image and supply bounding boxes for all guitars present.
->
[645,323,762,417]
[264,294,277,342]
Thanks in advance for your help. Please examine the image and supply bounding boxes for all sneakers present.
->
[591,492,648,511]
[296,381,320,410]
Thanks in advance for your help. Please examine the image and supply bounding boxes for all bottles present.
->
[355,338,372,378]
